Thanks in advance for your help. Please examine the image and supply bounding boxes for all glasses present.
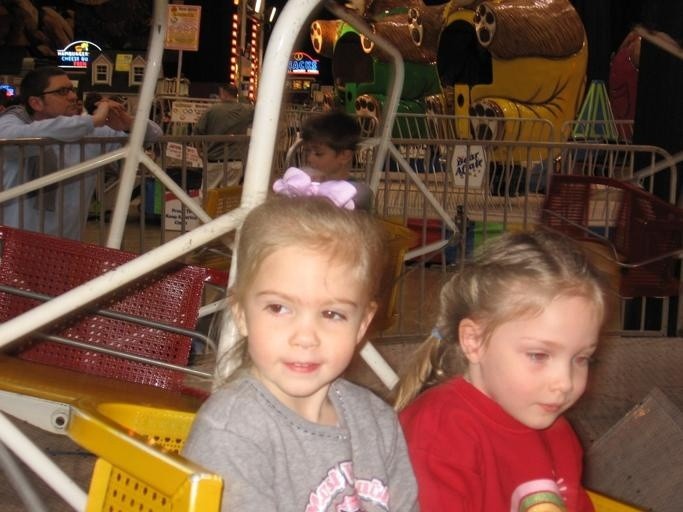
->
[36,86,77,95]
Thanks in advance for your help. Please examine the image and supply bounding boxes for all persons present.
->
[191,83,253,199]
[186,168,420,510]
[0,69,163,248]
[393,226,607,510]
[299,111,372,212]
